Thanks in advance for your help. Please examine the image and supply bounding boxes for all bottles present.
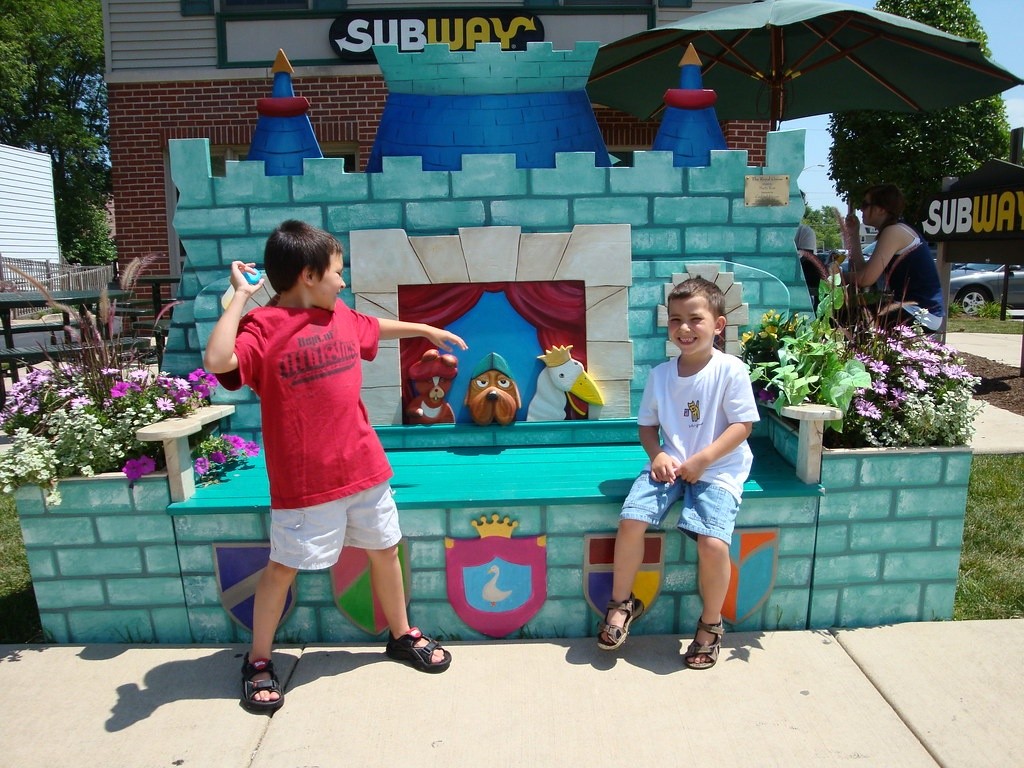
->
[221,268,262,311]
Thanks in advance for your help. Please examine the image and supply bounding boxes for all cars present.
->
[817,241,1024,317]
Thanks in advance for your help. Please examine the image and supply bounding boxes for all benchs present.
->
[0,320,150,412]
[92,298,177,373]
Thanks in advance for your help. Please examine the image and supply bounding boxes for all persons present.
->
[597,276,761,669]
[204,217,468,712]
[843,182,945,336]
[794,189,817,264]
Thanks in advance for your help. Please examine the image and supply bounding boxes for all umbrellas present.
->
[583,0,1024,131]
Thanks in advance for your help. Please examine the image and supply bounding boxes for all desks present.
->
[0,290,130,343]
[133,275,180,319]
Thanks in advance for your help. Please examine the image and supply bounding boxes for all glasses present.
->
[860,201,874,211]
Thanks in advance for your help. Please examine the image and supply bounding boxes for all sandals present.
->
[241,654,284,709]
[386,627,452,670]
[597,592,644,649]
[684,615,723,669]
